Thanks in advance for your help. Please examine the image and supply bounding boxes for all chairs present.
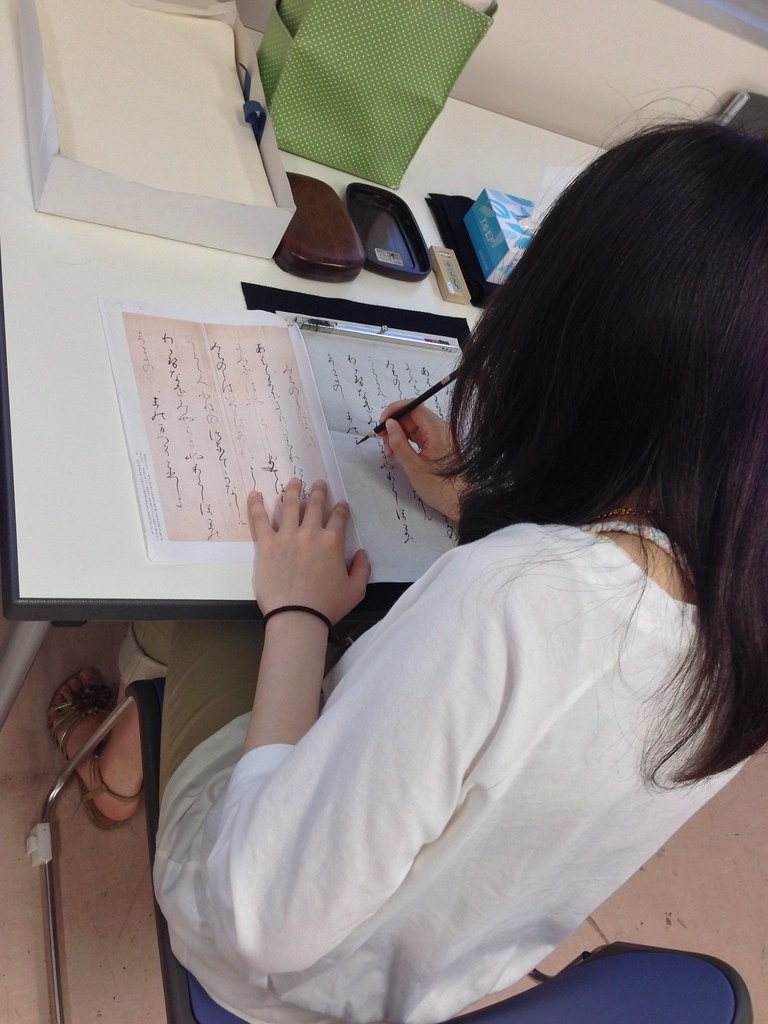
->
[27,679,755,1024]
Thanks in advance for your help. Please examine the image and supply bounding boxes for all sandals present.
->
[46,664,144,830]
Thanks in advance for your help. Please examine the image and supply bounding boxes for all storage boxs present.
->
[16,0,297,257]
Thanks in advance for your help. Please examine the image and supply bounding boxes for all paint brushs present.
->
[355,364,461,444]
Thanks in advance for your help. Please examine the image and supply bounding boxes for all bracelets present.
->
[263,606,332,630]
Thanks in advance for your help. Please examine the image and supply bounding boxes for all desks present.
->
[0,26,623,722]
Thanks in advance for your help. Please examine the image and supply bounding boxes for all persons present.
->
[48,121,768,1024]
